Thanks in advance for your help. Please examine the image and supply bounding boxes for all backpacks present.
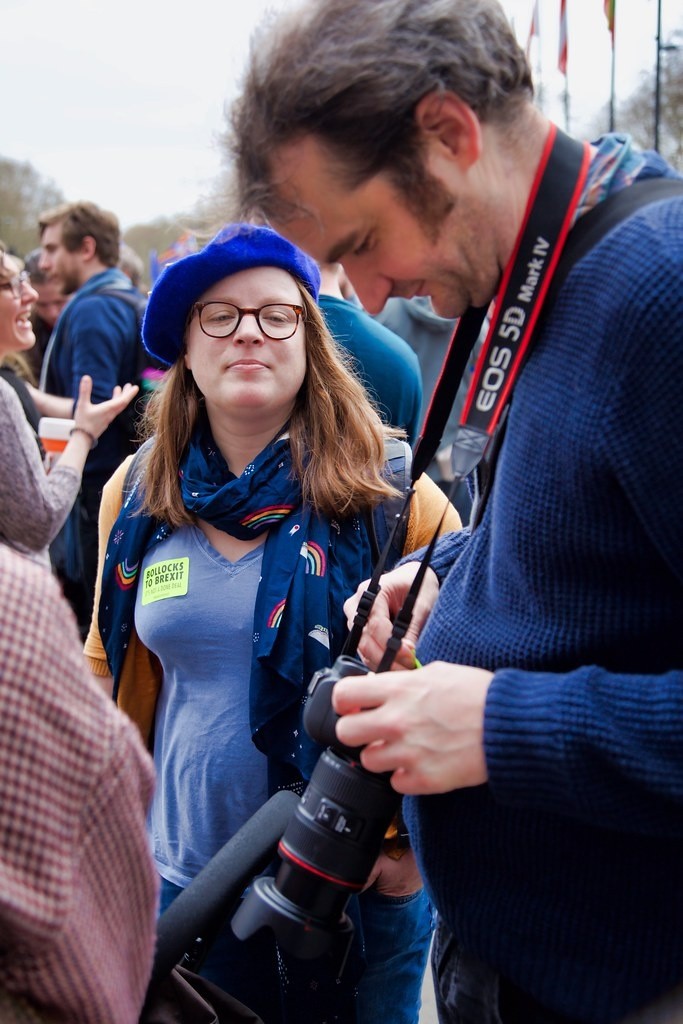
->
[93,288,162,452]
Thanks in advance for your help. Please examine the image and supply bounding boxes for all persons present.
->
[311,262,473,527]
[0,543,161,1024]
[117,243,145,286]
[23,250,77,382]
[82,227,465,1024]
[232,0,683,1023]
[0,247,140,555]
[39,201,147,643]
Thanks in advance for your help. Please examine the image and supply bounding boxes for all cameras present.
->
[231,655,407,987]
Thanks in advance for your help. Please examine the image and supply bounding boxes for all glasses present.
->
[0,271,31,297]
[183,301,307,341]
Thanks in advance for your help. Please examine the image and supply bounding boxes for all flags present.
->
[525,0,615,75]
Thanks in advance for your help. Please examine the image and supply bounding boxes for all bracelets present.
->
[70,428,96,449]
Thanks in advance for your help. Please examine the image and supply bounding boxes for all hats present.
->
[142,222,321,365]
